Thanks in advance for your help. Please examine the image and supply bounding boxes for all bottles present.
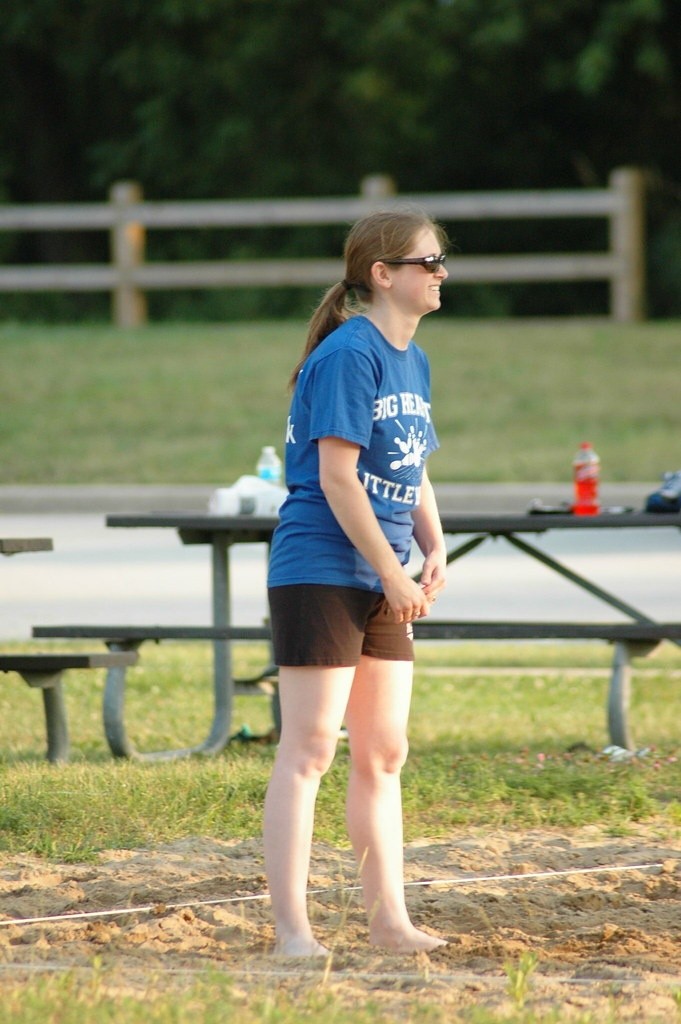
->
[572,441,601,517]
[212,488,285,515]
[257,445,283,487]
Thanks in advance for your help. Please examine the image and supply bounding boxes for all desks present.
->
[0,508,681,760]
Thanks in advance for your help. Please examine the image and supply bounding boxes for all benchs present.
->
[0,651,139,669]
[32,621,680,642]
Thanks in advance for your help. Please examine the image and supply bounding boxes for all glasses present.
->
[379,254,449,273]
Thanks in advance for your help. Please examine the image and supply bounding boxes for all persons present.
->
[260,212,451,967]
